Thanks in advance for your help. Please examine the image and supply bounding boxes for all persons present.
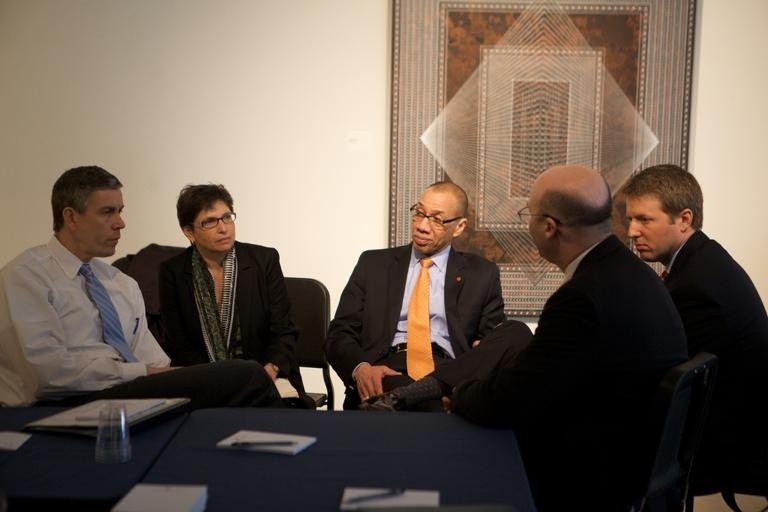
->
[623,165,767,496]
[0,165,283,409]
[322,181,534,411]
[441,164,691,512]
[159,185,315,408]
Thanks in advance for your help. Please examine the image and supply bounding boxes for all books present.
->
[23,398,191,437]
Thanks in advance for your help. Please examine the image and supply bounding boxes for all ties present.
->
[406,258,436,380]
[80,264,139,363]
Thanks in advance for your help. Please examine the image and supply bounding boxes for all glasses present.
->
[190,212,237,230]
[516,206,558,226]
[409,204,462,227]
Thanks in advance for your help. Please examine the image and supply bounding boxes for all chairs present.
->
[638,353,716,512]
[284,277,333,411]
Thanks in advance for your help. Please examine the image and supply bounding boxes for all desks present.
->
[0,408,535,512]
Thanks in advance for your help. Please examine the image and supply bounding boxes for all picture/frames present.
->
[386,0,697,318]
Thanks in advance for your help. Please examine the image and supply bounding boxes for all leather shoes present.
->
[358,393,397,412]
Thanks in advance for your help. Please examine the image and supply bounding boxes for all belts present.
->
[395,341,408,353]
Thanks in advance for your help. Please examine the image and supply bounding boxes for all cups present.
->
[95,401,131,465]
